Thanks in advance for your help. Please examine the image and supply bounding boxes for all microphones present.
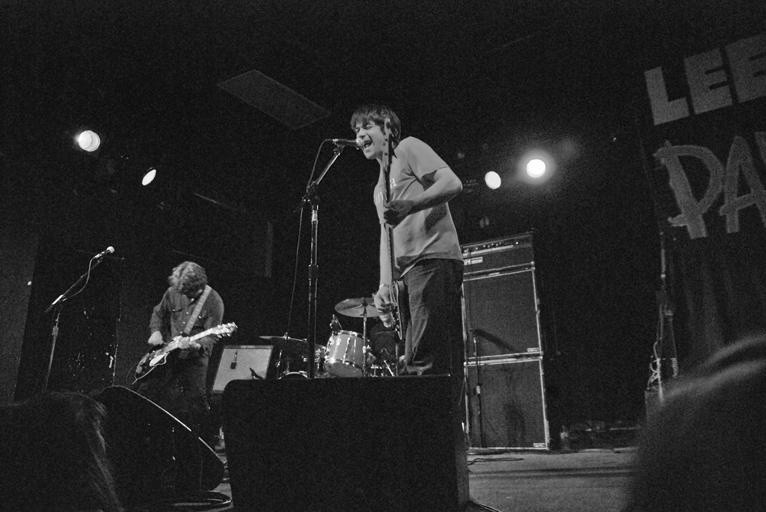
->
[231,352,237,369]
[332,137,364,150]
[94,246,115,259]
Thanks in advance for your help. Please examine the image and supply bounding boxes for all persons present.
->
[603,331,764,512]
[144,259,226,452]
[0,389,133,512]
[344,100,465,396]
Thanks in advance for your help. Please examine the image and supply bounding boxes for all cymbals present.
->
[259,335,325,358]
[334,295,379,318]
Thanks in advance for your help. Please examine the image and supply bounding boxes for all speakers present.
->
[90,385,224,512]
[220,373,470,512]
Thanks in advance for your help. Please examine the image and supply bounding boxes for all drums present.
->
[323,329,375,378]
[276,352,310,382]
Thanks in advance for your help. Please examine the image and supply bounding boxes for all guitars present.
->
[134,322,239,382]
[379,117,410,343]
